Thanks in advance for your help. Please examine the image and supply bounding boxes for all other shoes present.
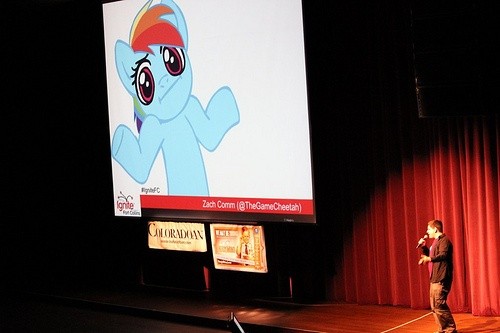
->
[434,329,457,333]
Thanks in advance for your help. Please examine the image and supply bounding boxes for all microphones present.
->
[416,234,428,250]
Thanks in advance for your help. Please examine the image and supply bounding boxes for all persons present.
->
[235,226,255,267]
[417,219,457,333]
